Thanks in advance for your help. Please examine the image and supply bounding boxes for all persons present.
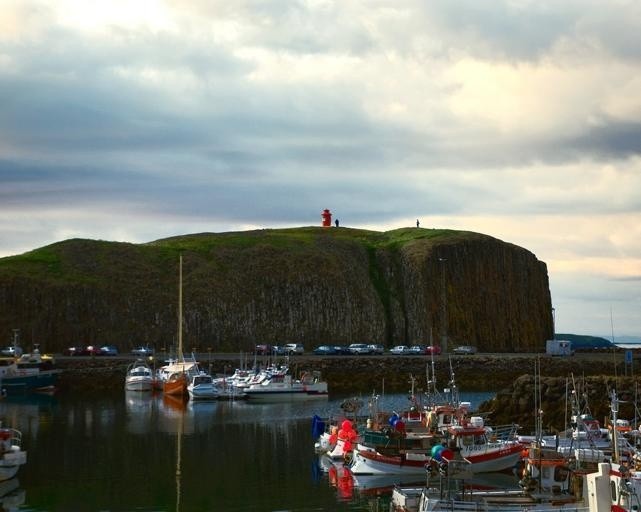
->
[417,219,421,227]
[335,219,340,227]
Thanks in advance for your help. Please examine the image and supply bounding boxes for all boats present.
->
[0,328,79,398]
[0,421,28,482]
[123,255,342,400]
[124,388,330,511]
[1,478,33,511]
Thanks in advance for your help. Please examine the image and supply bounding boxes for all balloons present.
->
[431,443,444,458]
[349,428,357,440]
[393,419,401,427]
[342,420,353,432]
[388,415,399,426]
[338,428,349,439]
[395,421,406,432]
[442,448,454,460]
[434,446,450,461]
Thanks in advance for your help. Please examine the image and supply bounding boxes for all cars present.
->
[63,342,120,356]
[0,346,23,356]
